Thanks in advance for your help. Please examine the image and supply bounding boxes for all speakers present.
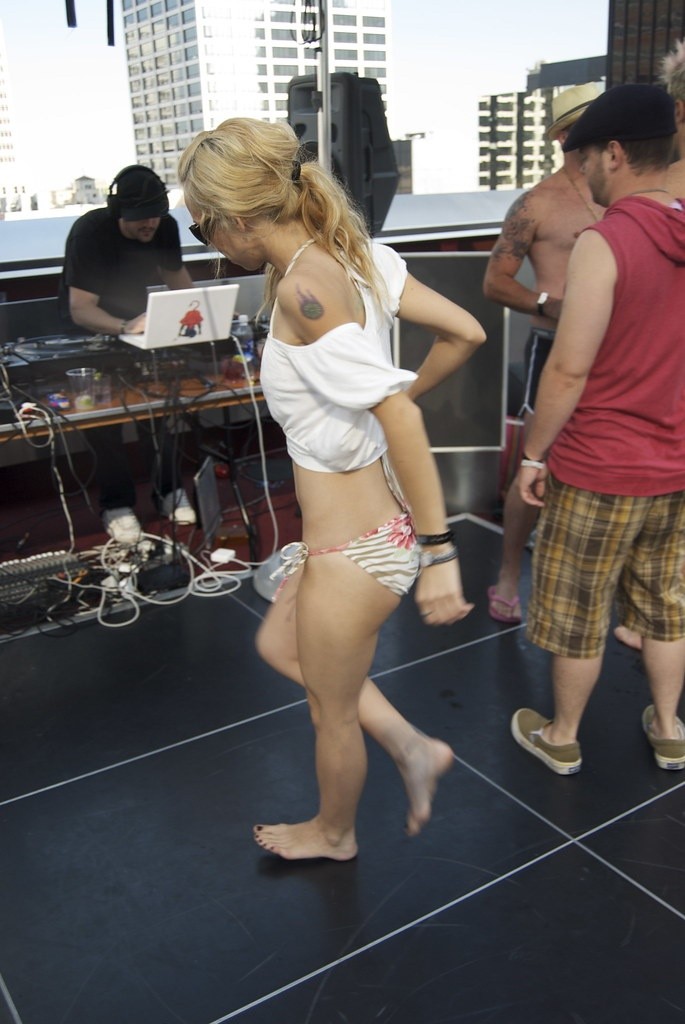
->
[287,72,400,237]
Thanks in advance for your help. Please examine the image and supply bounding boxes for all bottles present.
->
[233,314,255,379]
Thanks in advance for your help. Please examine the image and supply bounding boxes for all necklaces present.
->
[559,165,598,223]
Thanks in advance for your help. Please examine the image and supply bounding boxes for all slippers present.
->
[487,585,521,623]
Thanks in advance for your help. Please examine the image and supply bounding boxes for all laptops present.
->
[120,284,240,350]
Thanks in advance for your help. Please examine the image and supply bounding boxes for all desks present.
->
[0,361,266,571]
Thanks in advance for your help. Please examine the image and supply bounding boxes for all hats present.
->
[561,82,677,152]
[546,85,601,139]
[116,166,169,220]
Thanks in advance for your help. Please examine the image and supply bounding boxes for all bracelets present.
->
[121,318,129,334]
[420,546,459,567]
[520,451,545,470]
[537,292,548,317]
[414,528,454,547]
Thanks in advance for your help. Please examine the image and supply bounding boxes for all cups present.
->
[65,367,98,411]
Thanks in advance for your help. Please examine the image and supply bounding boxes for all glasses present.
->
[188,218,216,247]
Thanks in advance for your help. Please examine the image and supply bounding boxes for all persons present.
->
[179,117,486,860]
[483,34,685,647]
[48,165,240,542]
[515,78,685,775]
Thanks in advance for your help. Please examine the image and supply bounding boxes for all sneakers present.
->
[643,704,685,770]
[104,507,142,541]
[512,708,581,775]
[159,489,196,526]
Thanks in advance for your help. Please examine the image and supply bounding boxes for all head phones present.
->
[107,167,169,217]
